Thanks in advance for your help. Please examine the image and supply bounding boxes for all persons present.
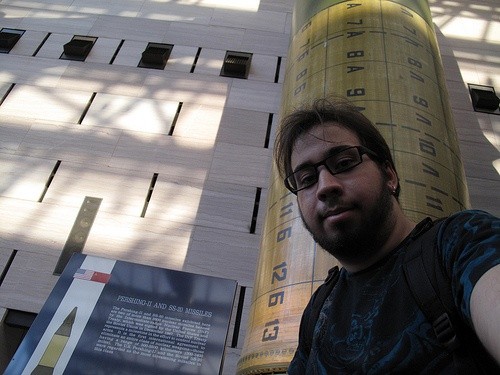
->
[272,95,499,375]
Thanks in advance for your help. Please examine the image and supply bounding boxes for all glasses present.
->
[284,145,382,193]
